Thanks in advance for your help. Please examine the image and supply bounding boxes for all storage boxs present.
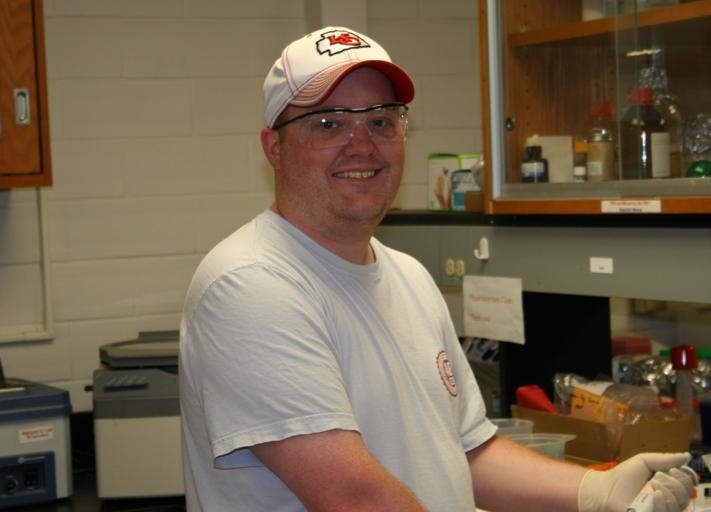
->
[509,403,693,467]
[427,152,483,211]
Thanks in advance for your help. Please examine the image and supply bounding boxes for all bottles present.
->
[519,145,550,184]
[571,85,674,182]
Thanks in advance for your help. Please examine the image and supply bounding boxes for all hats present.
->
[261,26,415,130]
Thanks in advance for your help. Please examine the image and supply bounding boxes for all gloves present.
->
[578,451,694,512]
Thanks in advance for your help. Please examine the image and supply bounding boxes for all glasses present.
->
[270,102,409,148]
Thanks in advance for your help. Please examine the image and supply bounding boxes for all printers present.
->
[92,328,187,500]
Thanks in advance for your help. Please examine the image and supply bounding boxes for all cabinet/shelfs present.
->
[478,0,710,215]
[0,1,53,189]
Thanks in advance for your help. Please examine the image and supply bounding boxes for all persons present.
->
[177,27,696,512]
